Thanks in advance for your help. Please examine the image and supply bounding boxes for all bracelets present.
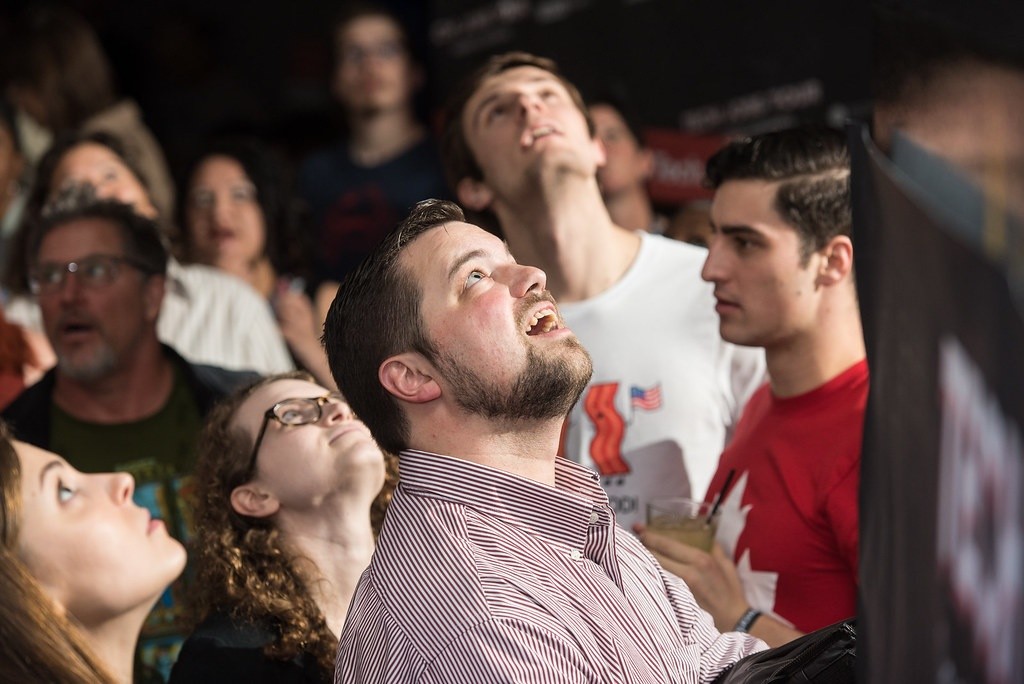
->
[732,607,763,633]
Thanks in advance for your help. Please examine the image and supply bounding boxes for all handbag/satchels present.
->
[706,611,860,684]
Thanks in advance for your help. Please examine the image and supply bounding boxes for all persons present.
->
[0,9,1022,684]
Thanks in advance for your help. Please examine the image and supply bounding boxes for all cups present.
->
[647,498,722,558]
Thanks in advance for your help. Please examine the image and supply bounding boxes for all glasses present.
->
[246,390,357,478]
[28,255,158,295]
